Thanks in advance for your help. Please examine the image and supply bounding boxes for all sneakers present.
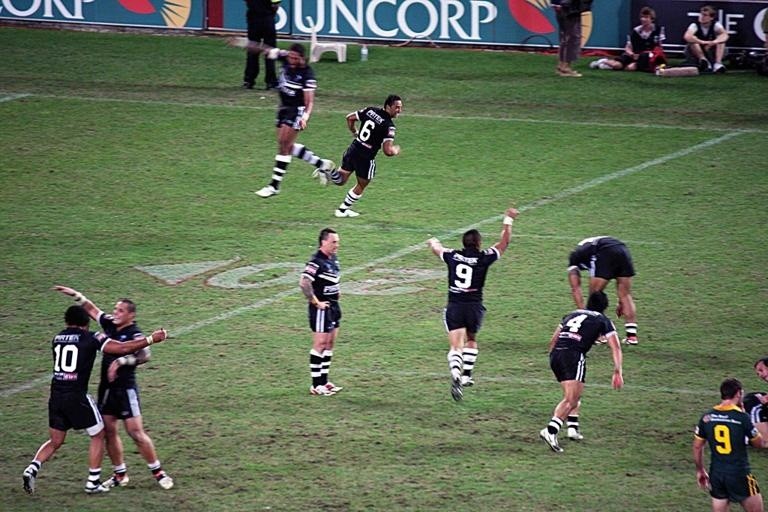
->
[266,81,279,89]
[620,336,638,345]
[699,57,713,72]
[335,208,360,218]
[568,428,584,440]
[590,58,614,70]
[85,468,174,494]
[23,466,36,495]
[312,159,336,189]
[555,66,582,77]
[539,426,563,453]
[451,368,463,403]
[254,185,280,198]
[243,80,254,90]
[713,63,726,74]
[459,374,476,387]
[310,380,342,397]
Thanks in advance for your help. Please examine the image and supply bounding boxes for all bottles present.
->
[360,43,368,61]
[654,64,665,77]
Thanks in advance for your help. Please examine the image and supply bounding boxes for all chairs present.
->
[307,16,347,64]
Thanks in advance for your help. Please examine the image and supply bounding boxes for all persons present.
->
[49,284,174,491]
[299,229,344,396]
[242,0,283,92]
[549,0,731,79]
[224,35,335,198]
[19,306,167,493]
[567,235,639,345]
[539,291,624,452]
[311,95,403,218]
[743,357,768,449]
[692,378,763,512]
[425,207,522,402]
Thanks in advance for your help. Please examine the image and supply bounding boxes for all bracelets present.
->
[307,294,320,306]
[352,130,358,138]
[503,216,514,225]
[145,335,154,346]
[300,112,311,122]
[116,354,135,365]
